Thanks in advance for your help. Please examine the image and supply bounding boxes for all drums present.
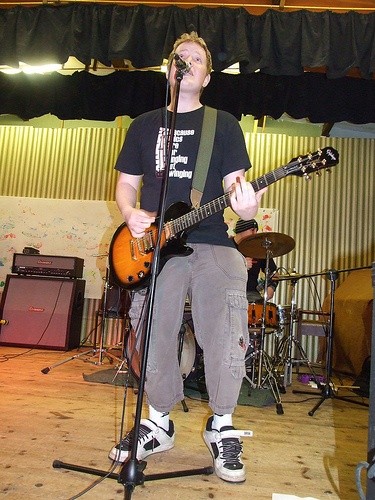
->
[248,301,279,334]
[122,312,196,385]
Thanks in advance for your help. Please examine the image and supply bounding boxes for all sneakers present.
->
[202,415,254,483]
[107,419,175,463]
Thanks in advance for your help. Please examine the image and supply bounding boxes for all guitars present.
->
[107,145,340,293]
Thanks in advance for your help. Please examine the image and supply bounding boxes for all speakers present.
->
[0,274,86,353]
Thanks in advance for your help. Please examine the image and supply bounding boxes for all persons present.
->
[233,217,279,302]
[109,32,269,483]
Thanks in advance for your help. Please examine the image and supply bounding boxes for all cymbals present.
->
[237,231,296,259]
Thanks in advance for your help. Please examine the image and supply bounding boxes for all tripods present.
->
[243,241,372,416]
[40,254,131,374]
[51,66,213,500]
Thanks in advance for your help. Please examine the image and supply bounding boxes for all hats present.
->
[233,217,259,234]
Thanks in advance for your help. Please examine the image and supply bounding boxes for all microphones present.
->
[173,53,191,74]
[0,319,9,325]
